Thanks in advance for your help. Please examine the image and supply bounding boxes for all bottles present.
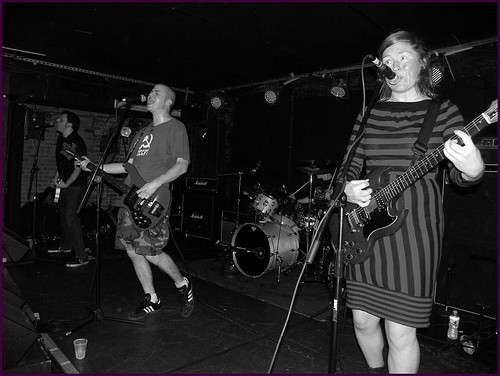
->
[447,310,460,339]
[460,335,474,354]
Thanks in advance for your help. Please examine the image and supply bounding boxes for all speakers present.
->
[180,193,223,240]
[434,169,497,321]
[220,211,250,244]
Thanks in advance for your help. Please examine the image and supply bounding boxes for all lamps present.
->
[263,88,279,104]
[428,60,444,84]
[330,77,347,98]
[210,94,226,109]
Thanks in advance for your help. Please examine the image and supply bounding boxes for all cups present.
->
[73,338,87,359]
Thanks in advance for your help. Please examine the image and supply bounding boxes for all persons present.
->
[46,110,90,267]
[336,30,485,373]
[289,173,336,204]
[77,83,194,321]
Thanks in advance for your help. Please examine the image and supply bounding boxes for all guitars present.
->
[58,141,172,231]
[47,174,64,206]
[327,96,499,266]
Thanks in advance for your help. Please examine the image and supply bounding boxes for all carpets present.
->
[178,258,343,323]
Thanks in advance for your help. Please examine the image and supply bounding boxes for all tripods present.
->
[65,104,146,337]
[281,174,317,274]
[3,131,64,267]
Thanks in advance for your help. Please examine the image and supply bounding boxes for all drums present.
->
[230,222,301,279]
[270,196,304,227]
[248,190,280,217]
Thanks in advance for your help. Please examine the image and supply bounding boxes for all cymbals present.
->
[288,158,327,174]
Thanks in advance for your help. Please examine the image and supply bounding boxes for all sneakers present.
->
[127,293,164,322]
[48,244,73,253]
[65,258,90,267]
[174,275,195,319]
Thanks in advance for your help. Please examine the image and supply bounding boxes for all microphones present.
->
[32,106,37,121]
[368,54,400,84]
[35,122,56,128]
[122,95,147,102]
